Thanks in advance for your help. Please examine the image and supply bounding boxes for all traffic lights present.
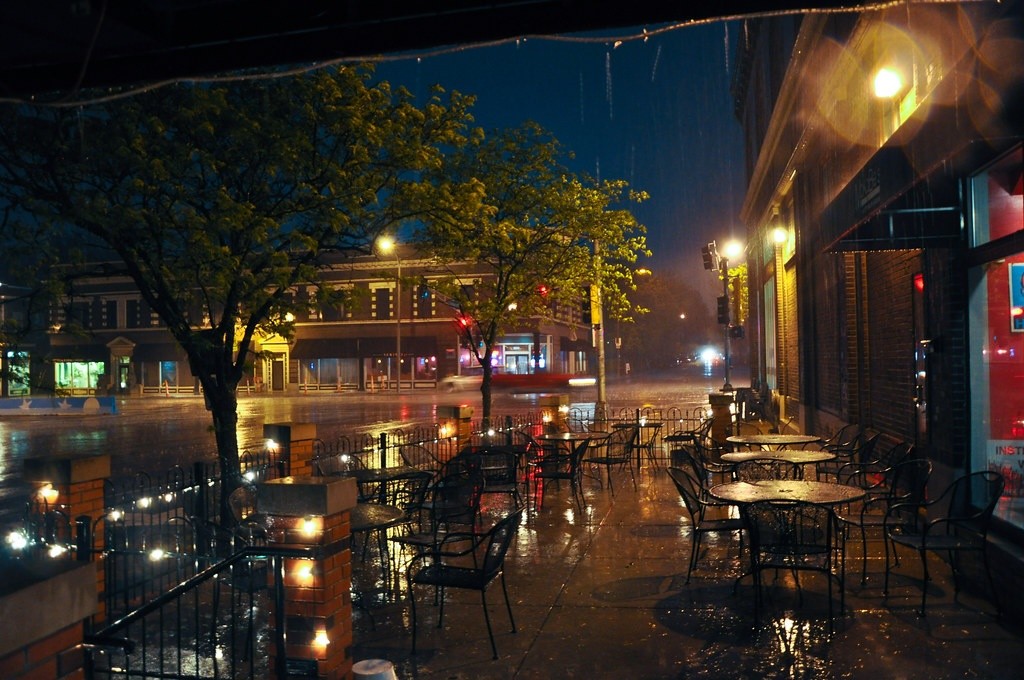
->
[538,286,549,297]
[459,316,471,347]
[716,297,726,325]
[700,245,712,270]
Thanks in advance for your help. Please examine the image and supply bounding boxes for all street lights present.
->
[874,64,933,455]
[375,233,403,399]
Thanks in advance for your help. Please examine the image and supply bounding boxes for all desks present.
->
[538,431,611,493]
[330,468,431,555]
[610,423,661,468]
[461,444,530,513]
[726,432,821,480]
[720,451,837,525]
[348,502,404,624]
[708,480,868,602]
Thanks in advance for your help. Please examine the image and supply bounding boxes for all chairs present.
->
[191,379,1012,680]
